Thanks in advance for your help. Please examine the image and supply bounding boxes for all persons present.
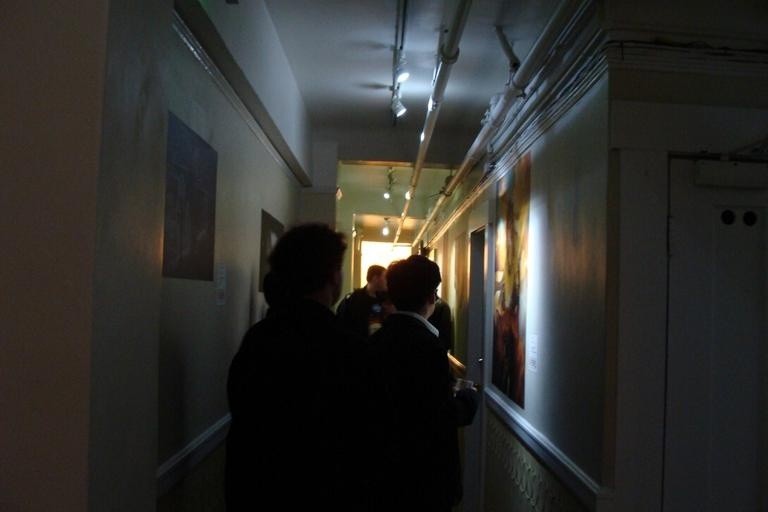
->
[217,220,481,511]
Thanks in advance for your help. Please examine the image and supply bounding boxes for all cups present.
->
[453,377,478,398]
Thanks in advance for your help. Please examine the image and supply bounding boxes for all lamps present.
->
[390,0,410,126]
